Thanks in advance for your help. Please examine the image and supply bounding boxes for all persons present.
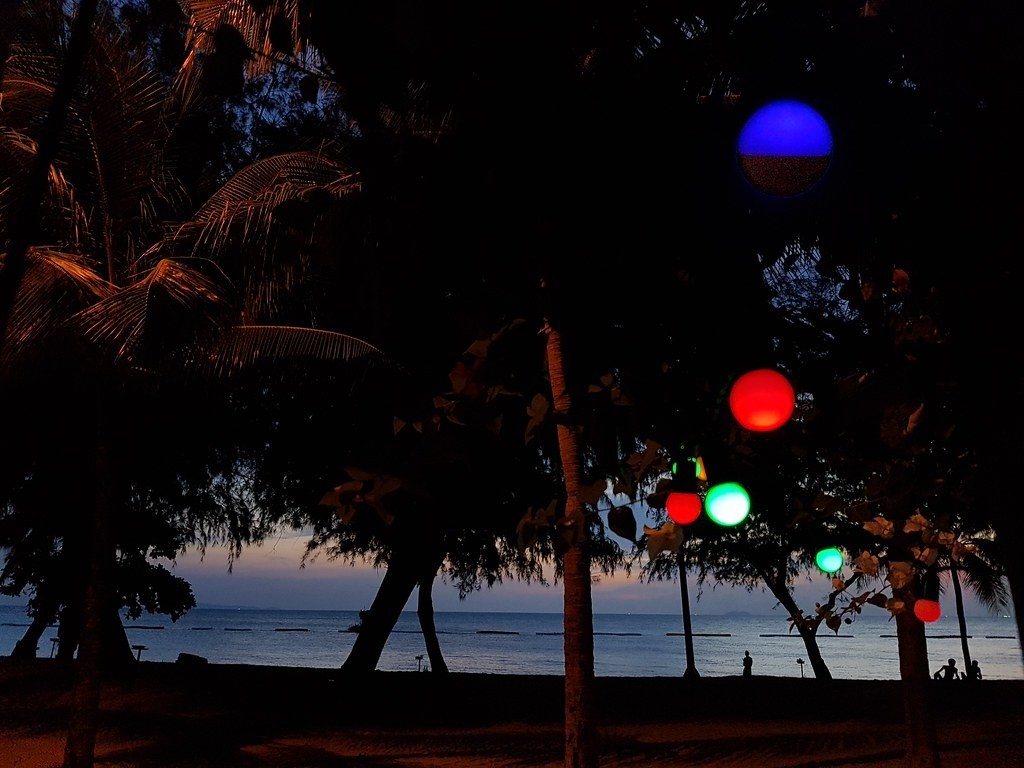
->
[742,650,753,676]
[934,658,960,680]
[961,660,982,680]
